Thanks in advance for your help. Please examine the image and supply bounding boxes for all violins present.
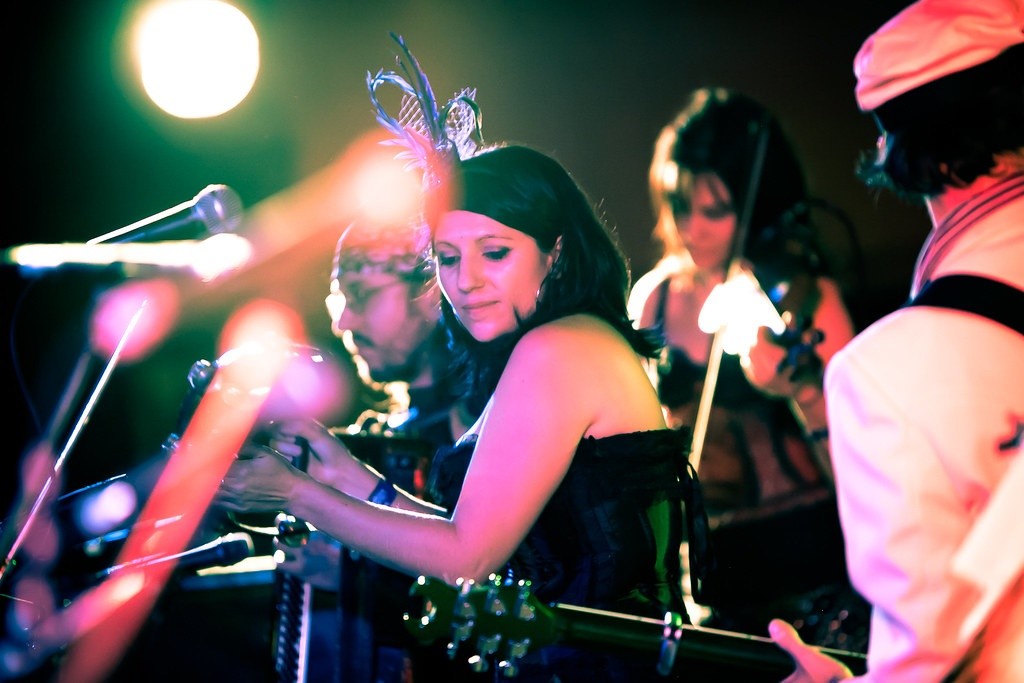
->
[693,256,844,487]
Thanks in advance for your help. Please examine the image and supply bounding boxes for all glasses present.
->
[324,273,409,321]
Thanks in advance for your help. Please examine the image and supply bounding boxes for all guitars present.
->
[404,568,866,683]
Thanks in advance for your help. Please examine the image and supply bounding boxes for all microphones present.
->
[90,534,254,588]
[75,184,242,265]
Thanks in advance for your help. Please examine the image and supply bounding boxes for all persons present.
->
[171,0,1024,683]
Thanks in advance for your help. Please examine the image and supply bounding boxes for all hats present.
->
[852,0,1024,112]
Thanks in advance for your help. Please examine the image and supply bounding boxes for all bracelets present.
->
[366,477,397,506]
